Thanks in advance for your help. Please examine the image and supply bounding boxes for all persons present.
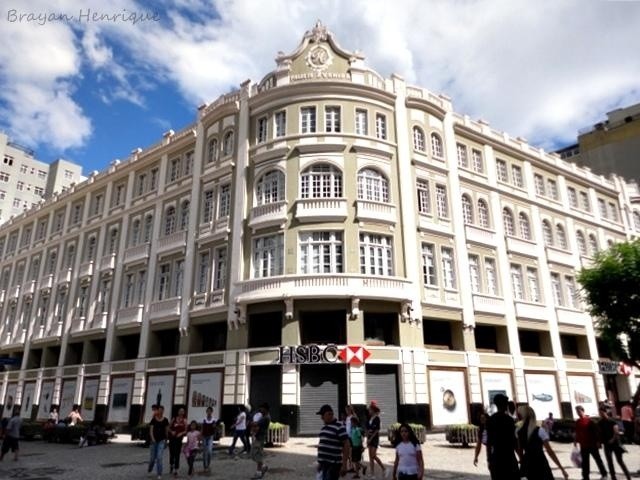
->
[42,404,84,426]
[0,409,24,462]
[472,382,640,480]
[314,399,425,480]
[146,403,270,480]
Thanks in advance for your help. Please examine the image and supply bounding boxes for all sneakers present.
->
[347,465,390,480]
[146,468,211,480]
[250,464,268,480]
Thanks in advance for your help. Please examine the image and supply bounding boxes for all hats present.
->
[314,403,333,415]
